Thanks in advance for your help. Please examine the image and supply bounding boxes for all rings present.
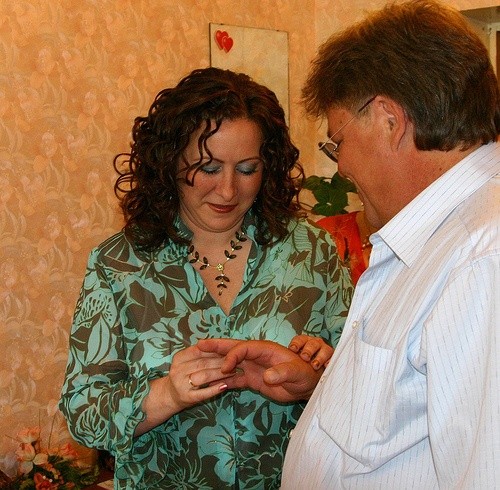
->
[189,372,199,387]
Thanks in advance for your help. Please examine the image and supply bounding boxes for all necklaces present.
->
[165,219,257,299]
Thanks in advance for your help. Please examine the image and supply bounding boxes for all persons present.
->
[58,66,355,490]
[192,0,500,490]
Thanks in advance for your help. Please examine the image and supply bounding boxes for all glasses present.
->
[319,94,380,162]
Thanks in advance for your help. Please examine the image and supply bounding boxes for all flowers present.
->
[0,427,100,490]
